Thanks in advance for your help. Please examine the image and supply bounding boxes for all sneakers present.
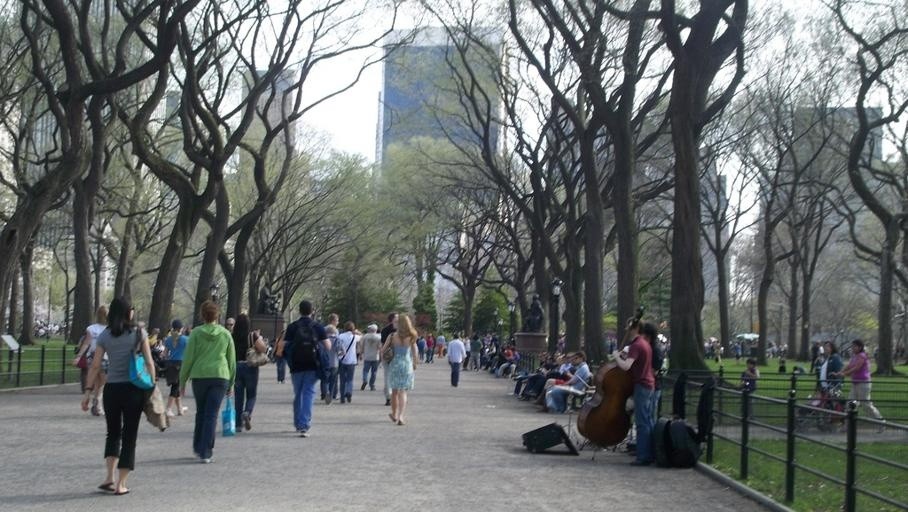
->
[389,413,398,421]
[320,391,352,404]
[630,459,649,465]
[178,406,188,415]
[386,400,391,405]
[300,429,310,437]
[242,412,251,431]
[362,381,368,390]
[235,426,242,432]
[200,457,216,463]
[398,422,406,425]
[166,408,175,416]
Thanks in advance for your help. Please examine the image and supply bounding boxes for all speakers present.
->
[522,423,579,455]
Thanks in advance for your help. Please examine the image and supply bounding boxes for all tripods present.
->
[562,397,580,445]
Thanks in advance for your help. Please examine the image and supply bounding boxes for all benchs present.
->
[525,374,593,411]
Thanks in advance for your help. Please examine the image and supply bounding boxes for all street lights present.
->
[209,281,220,302]
[839,327,844,357]
[548,274,565,356]
[493,305,499,335]
[508,300,517,345]
[439,310,443,334]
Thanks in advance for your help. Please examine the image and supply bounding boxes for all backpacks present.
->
[289,319,319,374]
[651,417,702,467]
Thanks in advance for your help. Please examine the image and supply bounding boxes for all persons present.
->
[71,301,266,496]
[260,283,277,314]
[415,330,519,387]
[705,336,885,433]
[273,301,419,437]
[516,318,662,468]
[523,294,543,331]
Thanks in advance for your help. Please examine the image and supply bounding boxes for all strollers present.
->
[790,374,849,435]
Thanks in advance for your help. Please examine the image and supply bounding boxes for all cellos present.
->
[576,308,644,446]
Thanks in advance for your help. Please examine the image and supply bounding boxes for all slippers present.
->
[99,482,116,491]
[115,488,131,495]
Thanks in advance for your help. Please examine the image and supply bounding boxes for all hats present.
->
[173,319,184,328]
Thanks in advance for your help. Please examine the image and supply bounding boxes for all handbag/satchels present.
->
[129,350,154,390]
[246,346,270,368]
[384,348,393,363]
[222,397,237,435]
[73,354,87,369]
